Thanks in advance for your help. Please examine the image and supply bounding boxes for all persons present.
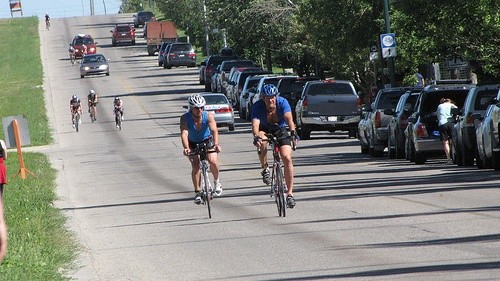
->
[70,95,82,127]
[45,14,50,29]
[81,45,87,56]
[180,93,222,204]
[87,90,97,120]
[251,84,299,206]
[114,97,123,128]
[69,46,75,63]
[0,140,7,260]
[437,98,458,164]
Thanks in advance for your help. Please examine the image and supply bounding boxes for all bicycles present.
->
[47,21,50,30]
[72,108,80,132]
[89,102,95,122]
[185,143,220,218]
[116,109,123,131]
[258,134,299,217]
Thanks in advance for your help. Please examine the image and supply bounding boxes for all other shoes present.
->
[447,159,453,165]
[214,182,222,197]
[286,197,296,206]
[262,172,270,184]
[194,193,202,204]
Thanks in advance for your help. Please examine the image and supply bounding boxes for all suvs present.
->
[69,35,99,58]
[110,24,136,47]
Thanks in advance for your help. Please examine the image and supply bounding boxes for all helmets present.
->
[188,93,206,108]
[72,94,77,99]
[261,83,279,97]
[89,90,94,94]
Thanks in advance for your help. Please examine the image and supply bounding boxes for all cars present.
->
[157,42,171,66]
[167,42,197,69]
[79,53,110,78]
[383,91,423,161]
[291,79,365,140]
[183,94,235,130]
[450,84,500,170]
[162,44,172,69]
[356,84,420,156]
[199,48,308,123]
[132,11,156,27]
[405,82,478,165]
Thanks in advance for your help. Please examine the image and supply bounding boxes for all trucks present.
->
[144,22,178,56]
